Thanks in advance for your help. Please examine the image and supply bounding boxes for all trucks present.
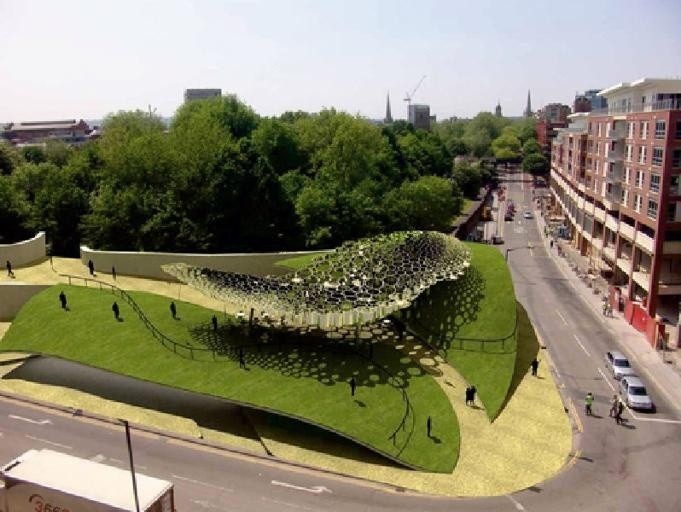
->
[0,447,175,512]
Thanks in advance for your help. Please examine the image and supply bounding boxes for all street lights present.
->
[505,244,534,261]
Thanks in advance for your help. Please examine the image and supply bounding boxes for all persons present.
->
[110,301,120,320]
[467,384,478,406]
[86,259,94,276]
[600,300,614,319]
[608,394,619,418]
[168,301,177,319]
[5,260,14,277]
[347,377,357,397]
[614,401,626,425]
[57,290,67,309]
[213,313,219,334]
[584,390,595,416]
[530,358,540,376]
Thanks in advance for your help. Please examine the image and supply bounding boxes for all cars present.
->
[504,201,513,222]
[619,374,653,411]
[605,350,634,379]
[523,210,532,218]
